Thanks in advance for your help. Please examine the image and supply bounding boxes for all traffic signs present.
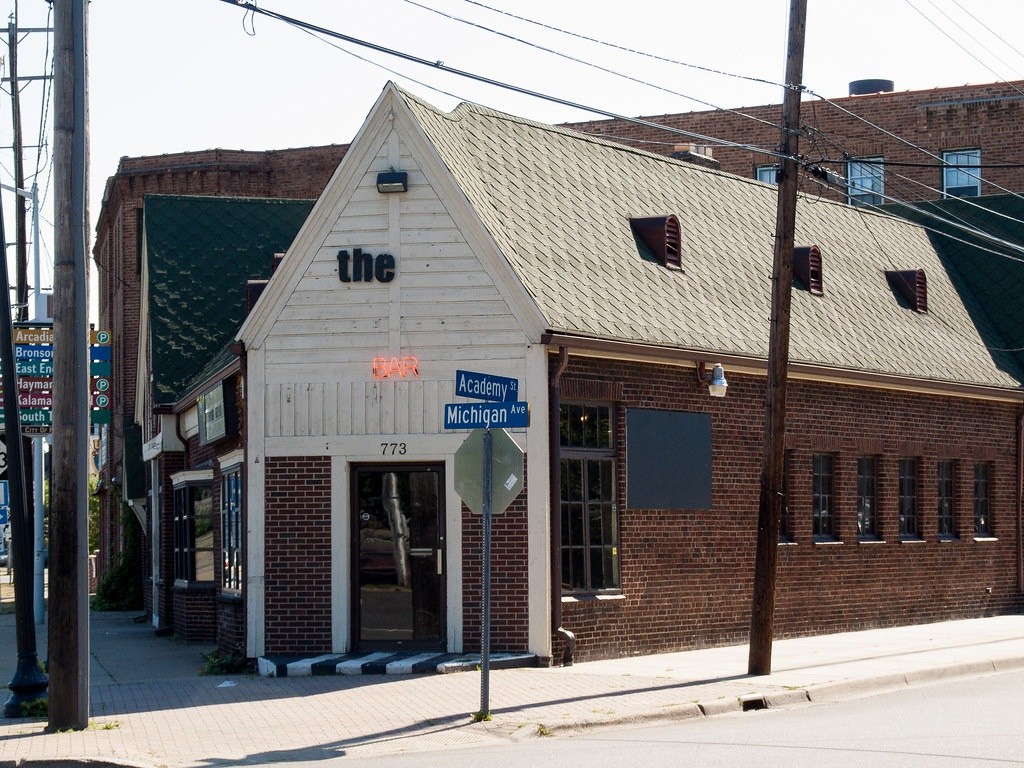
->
[443,369,531,433]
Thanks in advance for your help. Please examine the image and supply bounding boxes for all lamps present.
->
[694,360,728,397]
[377,172,408,194]
